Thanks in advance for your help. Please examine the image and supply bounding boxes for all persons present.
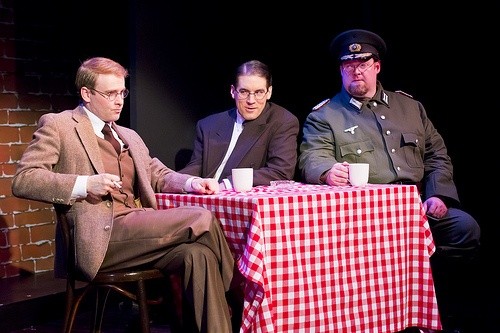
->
[300,29,481,333]
[11,57,246,333]
[176,60,298,191]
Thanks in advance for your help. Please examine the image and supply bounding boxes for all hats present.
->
[329,29,386,59]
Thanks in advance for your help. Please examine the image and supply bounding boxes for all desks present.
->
[155,181,443,333]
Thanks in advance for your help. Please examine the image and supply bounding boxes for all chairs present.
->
[51,203,182,333]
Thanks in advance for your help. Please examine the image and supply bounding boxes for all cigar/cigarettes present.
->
[113,181,122,189]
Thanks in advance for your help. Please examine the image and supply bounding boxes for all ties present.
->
[101,124,121,156]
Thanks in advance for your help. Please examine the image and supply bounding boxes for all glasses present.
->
[235,89,268,99]
[87,88,129,101]
[342,63,376,72]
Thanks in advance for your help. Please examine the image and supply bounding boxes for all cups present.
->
[231,168,254,193]
[344,163,369,187]
